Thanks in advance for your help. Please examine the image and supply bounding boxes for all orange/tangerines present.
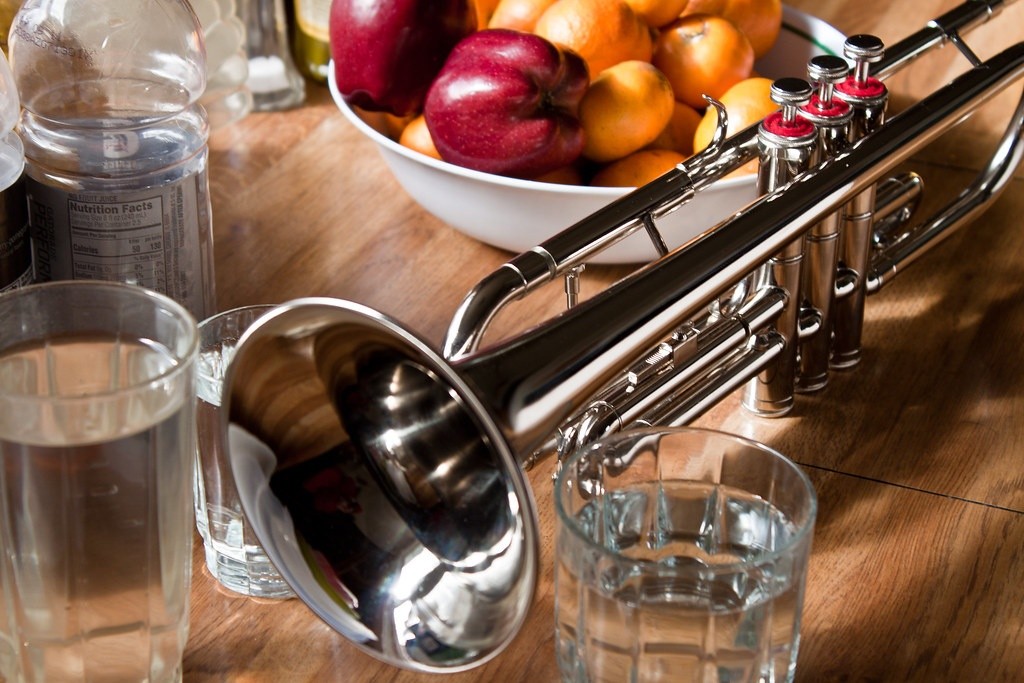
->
[389,0,792,188]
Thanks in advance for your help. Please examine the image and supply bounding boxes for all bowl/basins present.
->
[327,5,856,263]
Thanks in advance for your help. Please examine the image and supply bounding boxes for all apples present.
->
[329,0,479,118]
[423,28,590,178]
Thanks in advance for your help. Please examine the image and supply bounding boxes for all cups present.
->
[553,427,817,683]
[0,280,199,683]
[197,305,300,600]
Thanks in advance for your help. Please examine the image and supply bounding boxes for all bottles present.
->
[8,0,216,320]
[233,0,306,110]
[0,48,35,295]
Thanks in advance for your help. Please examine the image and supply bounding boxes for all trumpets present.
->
[218,0,1024,672]
